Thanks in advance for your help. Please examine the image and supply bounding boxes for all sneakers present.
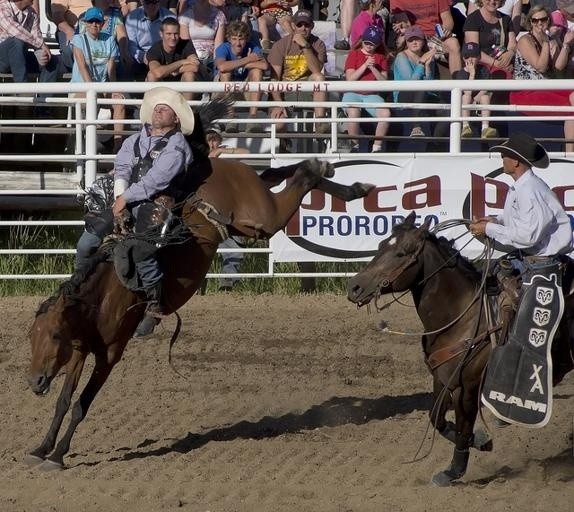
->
[494,418,511,428]
[225,116,240,133]
[410,127,426,137]
[260,39,274,49]
[245,115,257,133]
[113,138,123,153]
[481,127,496,138]
[315,123,331,141]
[334,40,350,50]
[461,126,473,137]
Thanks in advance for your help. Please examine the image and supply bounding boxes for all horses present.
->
[23,84,376,472]
[348,211,574,487]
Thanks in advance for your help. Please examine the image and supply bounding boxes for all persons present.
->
[468,131,574,428]
[76,86,195,337]
[205,129,251,291]
[1,1,573,152]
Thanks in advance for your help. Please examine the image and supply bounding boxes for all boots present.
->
[136,283,161,335]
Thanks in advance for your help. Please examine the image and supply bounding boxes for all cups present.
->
[34,50,48,66]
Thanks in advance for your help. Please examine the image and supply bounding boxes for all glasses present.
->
[532,17,548,23]
[296,22,310,28]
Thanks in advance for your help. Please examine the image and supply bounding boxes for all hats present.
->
[489,134,550,168]
[140,87,195,136]
[205,124,222,136]
[404,26,424,41]
[292,9,313,26]
[462,42,480,59]
[362,25,382,47]
[81,7,104,22]
[551,12,567,28]
